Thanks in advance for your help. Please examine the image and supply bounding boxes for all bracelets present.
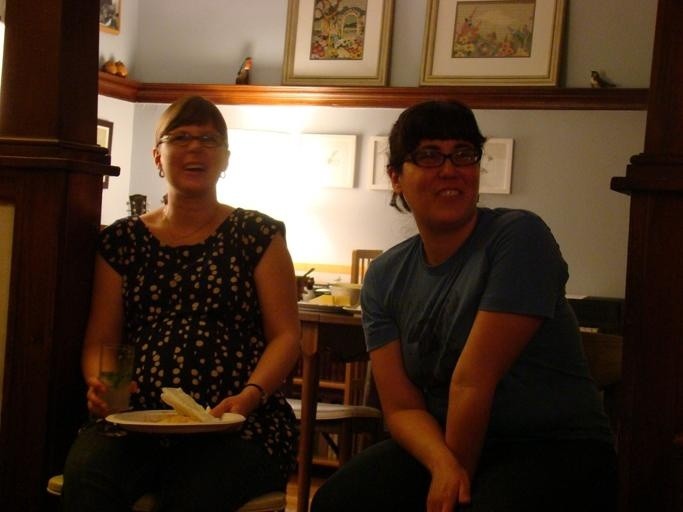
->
[244,383,268,410]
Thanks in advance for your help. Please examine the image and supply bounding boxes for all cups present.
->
[98,344,135,423]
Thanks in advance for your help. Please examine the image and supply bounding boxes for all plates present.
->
[104,410,246,434]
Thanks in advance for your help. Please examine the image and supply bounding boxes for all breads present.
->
[159,387,215,424]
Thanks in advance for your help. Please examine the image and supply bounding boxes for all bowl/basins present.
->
[329,283,363,308]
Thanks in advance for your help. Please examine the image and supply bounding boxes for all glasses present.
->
[156,132,222,148]
[400,146,482,168]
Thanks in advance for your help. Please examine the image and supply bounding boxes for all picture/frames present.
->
[280,0,394,87]
[369,134,515,194]
[97,118,113,190]
[99,0,121,36]
[420,0,565,87]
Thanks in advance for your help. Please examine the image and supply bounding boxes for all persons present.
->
[70,94,317,511]
[306,98,624,512]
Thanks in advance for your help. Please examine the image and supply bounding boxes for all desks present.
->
[296,309,363,512]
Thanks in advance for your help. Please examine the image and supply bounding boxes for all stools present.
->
[45,474,287,512]
